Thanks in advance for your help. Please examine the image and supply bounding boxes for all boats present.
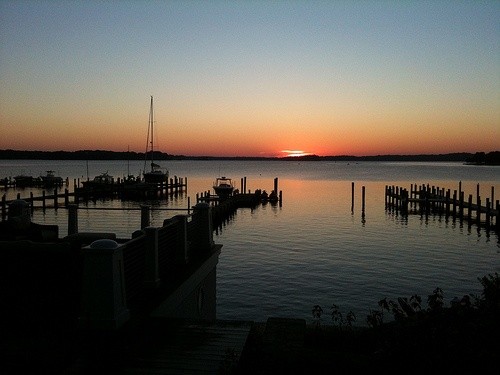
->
[74,96,189,200]
[0,168,64,191]
[194,174,283,210]
